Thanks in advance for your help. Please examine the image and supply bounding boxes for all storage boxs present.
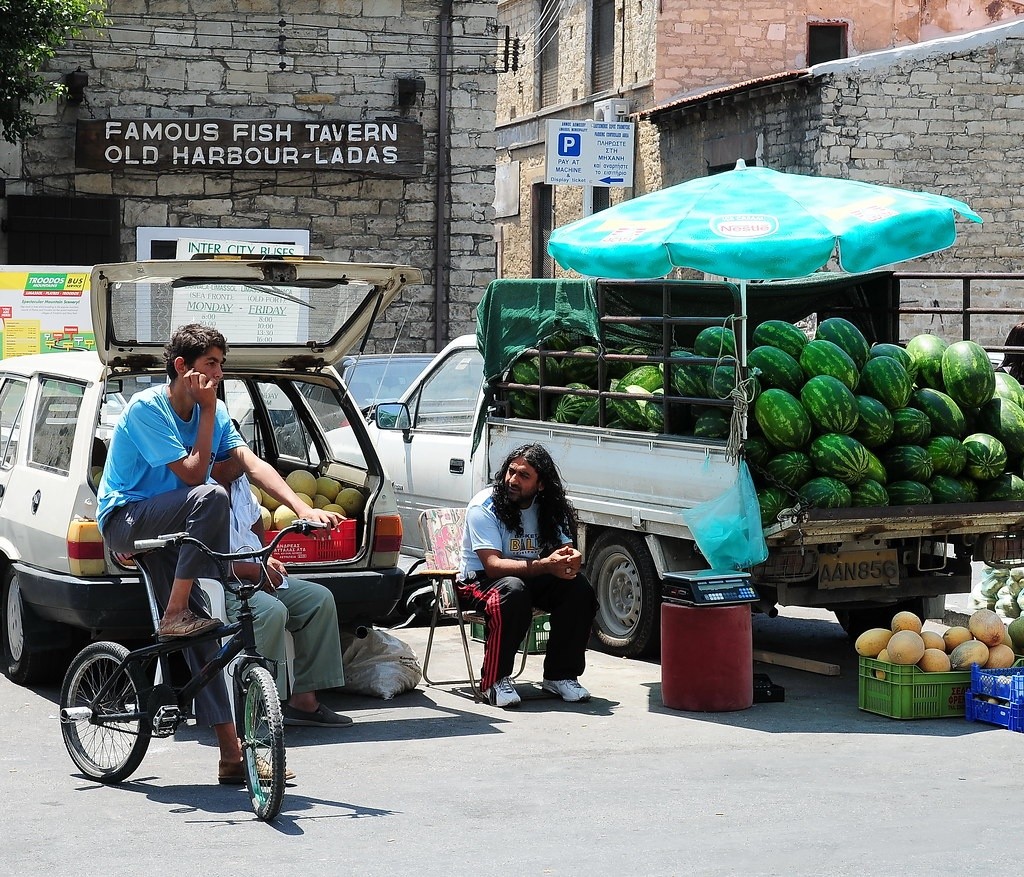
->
[859,654,1024,721]
[964,662,1024,732]
[260,518,357,562]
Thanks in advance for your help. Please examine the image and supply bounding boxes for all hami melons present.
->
[91,466,104,485]
[854,605,1015,678]
[246,470,365,532]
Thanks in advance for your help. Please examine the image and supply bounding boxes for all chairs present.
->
[416,507,553,702]
[36,396,82,444]
[350,383,374,406]
[375,375,405,400]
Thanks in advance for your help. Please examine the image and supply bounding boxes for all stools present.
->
[660,602,754,710]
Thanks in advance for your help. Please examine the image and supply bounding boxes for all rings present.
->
[566,568,571,573]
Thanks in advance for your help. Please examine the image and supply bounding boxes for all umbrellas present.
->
[545,157,983,441]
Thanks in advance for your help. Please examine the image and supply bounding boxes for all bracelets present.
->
[527,561,532,574]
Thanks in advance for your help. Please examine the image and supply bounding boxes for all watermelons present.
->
[502,317,1024,528]
[1008,615,1024,657]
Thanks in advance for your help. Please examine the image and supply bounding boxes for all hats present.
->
[215,418,247,463]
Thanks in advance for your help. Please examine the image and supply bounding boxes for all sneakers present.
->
[158,609,224,642]
[218,760,296,784]
[283,703,353,728]
[542,679,590,702]
[482,677,521,707]
[256,725,270,740]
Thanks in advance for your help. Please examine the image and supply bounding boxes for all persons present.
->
[96,324,347,784]
[202,418,353,728]
[455,443,597,707]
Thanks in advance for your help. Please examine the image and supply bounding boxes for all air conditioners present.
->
[593,97,630,124]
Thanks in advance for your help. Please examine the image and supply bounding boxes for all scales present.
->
[661,568,761,607]
